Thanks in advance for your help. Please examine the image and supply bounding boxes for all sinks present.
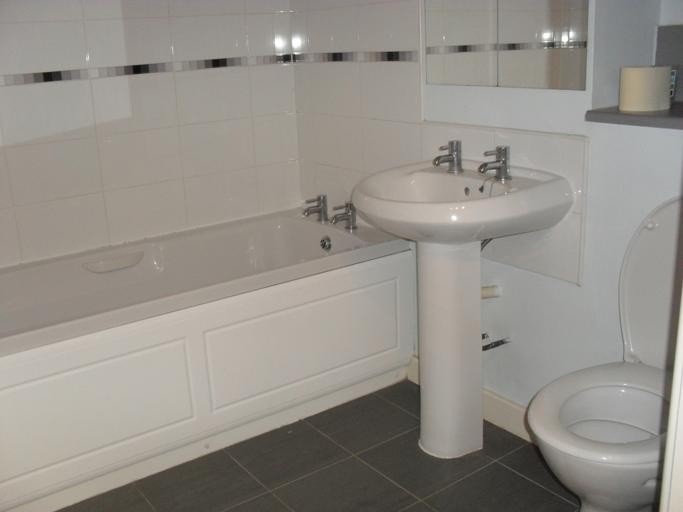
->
[350,156,574,243]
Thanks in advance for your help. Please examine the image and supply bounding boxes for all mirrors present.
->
[422,1,589,96]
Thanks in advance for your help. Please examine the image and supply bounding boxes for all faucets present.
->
[433,140,465,175]
[478,146,509,180]
[330,202,358,231]
[303,195,329,223]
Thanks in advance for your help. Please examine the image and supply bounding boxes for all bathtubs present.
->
[0,201,411,503]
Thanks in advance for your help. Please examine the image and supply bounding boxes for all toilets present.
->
[525,198,683,511]
